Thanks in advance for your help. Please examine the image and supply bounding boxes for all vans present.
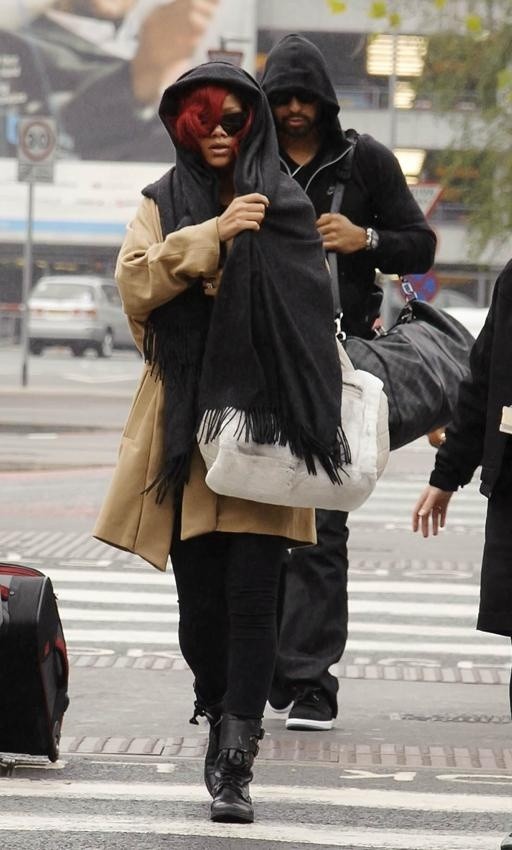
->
[385,286,478,339]
[21,269,140,360]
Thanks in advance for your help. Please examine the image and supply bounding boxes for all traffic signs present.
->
[16,114,57,165]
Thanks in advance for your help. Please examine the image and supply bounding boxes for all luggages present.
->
[0,562,70,762]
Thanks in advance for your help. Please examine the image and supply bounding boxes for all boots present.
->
[190,700,265,823]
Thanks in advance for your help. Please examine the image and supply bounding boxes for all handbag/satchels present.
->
[338,299,475,449]
[197,297,390,511]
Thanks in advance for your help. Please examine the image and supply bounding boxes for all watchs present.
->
[366,228,379,252]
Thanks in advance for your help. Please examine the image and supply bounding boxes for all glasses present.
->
[192,110,248,136]
[268,89,315,105]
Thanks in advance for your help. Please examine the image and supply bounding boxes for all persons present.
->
[90,61,351,821]
[413,257,512,850]
[256,34,435,730]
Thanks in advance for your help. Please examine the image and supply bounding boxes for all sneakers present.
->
[267,683,337,730]
